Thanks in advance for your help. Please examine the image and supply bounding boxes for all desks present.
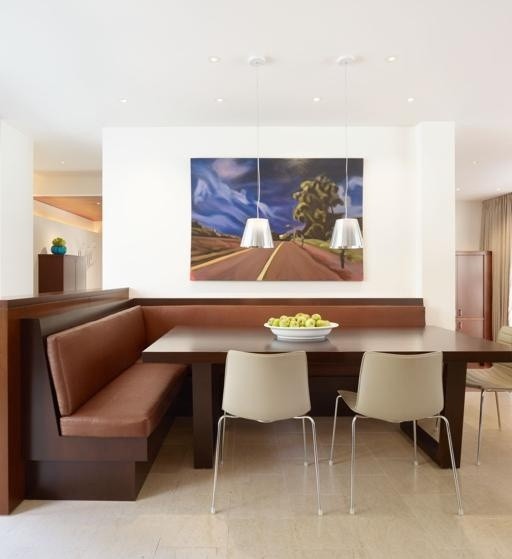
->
[144,323,511,473]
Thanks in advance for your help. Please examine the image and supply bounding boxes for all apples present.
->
[268,313,330,327]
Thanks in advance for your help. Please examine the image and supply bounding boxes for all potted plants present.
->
[50,237,67,254]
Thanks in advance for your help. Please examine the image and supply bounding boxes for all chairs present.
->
[328,351,466,515]
[208,351,324,518]
[464,326,512,467]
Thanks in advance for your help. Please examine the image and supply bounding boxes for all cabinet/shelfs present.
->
[38,253,86,294]
[455,250,493,369]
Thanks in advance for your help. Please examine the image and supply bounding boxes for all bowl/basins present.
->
[263,321,339,343]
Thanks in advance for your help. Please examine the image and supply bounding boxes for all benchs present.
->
[19,295,425,502]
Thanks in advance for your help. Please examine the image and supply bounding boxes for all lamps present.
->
[329,55,368,253]
[238,55,274,251]
